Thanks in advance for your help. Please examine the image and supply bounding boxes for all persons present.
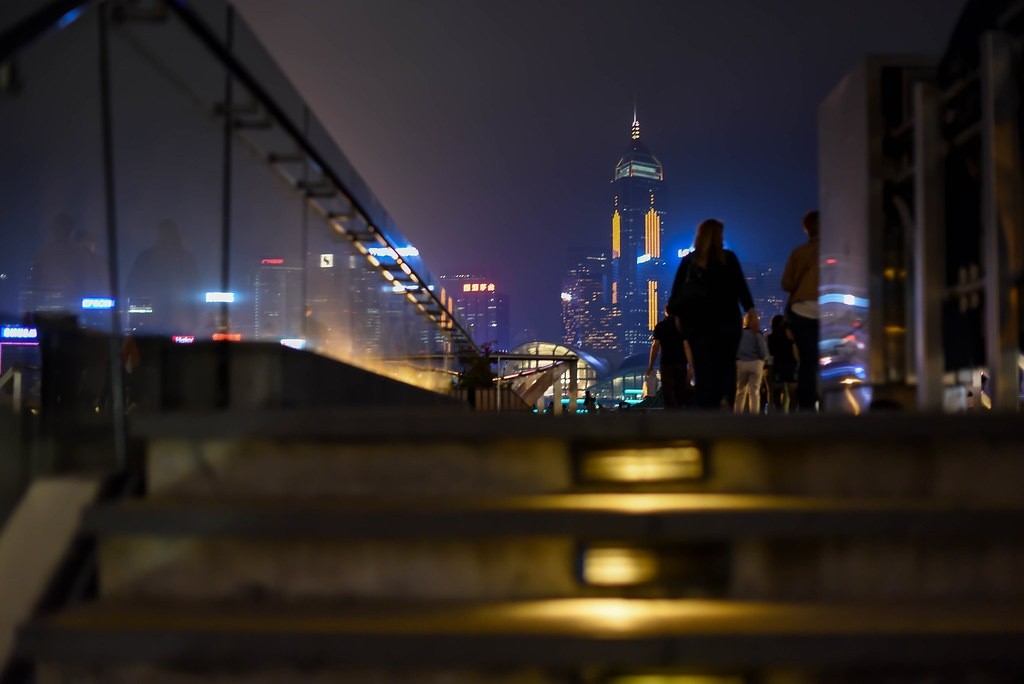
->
[643,210,822,416]
[583,391,595,413]
